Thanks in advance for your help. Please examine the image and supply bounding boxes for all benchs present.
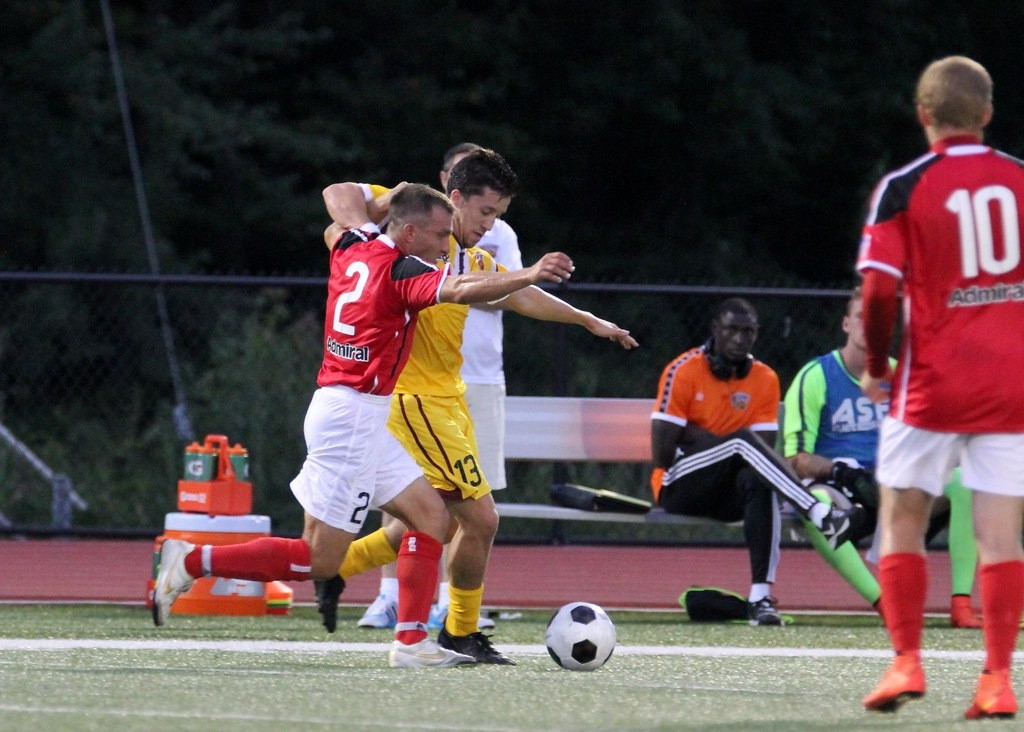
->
[495,394,790,526]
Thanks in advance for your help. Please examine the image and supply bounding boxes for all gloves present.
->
[832,457,867,499]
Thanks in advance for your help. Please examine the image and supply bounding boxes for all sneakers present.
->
[312,573,346,633]
[358,595,398,628]
[429,604,494,630]
[950,606,986,627]
[438,614,517,666]
[747,594,782,626]
[815,500,866,550]
[149,535,195,627]
[965,669,1018,718]
[864,655,925,710]
[389,635,476,671]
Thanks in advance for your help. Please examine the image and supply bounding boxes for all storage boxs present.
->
[164,512,271,616]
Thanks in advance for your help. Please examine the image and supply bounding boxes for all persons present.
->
[153,182,575,670]
[323,151,639,663]
[783,287,987,630]
[350,144,524,627]
[649,298,864,627]
[854,55,1024,719]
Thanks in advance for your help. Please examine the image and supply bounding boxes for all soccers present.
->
[545,602,616,673]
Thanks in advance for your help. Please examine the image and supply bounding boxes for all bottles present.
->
[153,536,164,580]
[184,441,219,480]
[226,443,248,480]
